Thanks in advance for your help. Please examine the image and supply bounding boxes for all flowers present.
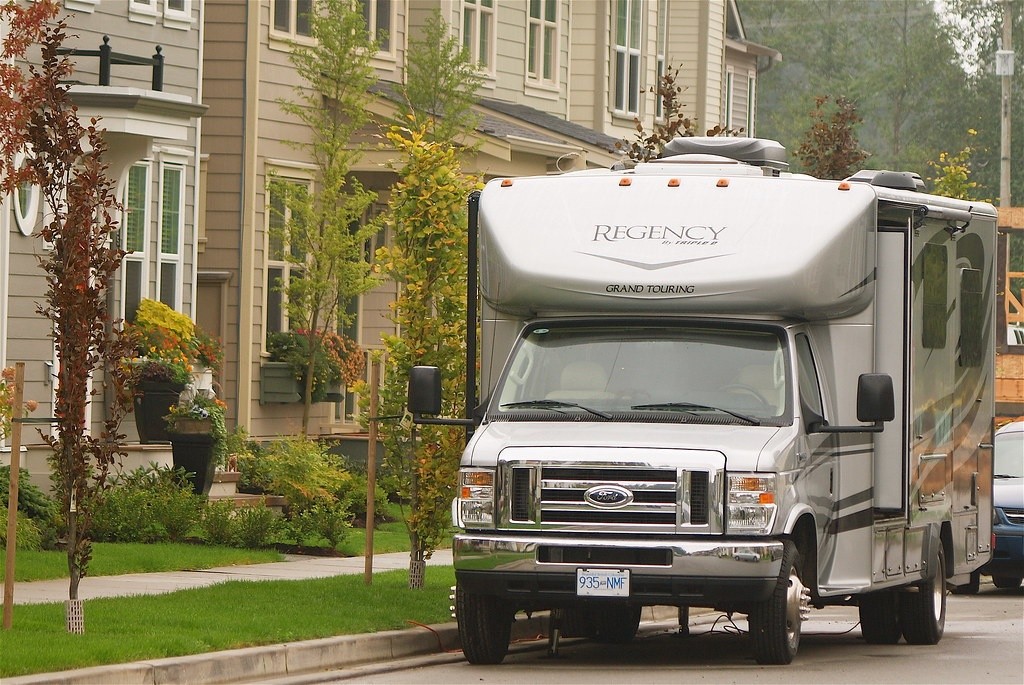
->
[116,297,225,385]
[161,368,229,466]
[0,366,40,441]
[266,327,366,399]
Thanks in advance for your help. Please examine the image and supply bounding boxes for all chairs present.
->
[545,361,615,400]
[732,365,778,406]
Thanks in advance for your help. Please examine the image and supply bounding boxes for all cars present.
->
[940,413,1023,598]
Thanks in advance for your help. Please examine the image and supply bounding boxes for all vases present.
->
[168,418,216,496]
[259,362,340,406]
[133,381,179,445]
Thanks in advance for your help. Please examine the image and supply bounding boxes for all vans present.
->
[407,135,999,665]
